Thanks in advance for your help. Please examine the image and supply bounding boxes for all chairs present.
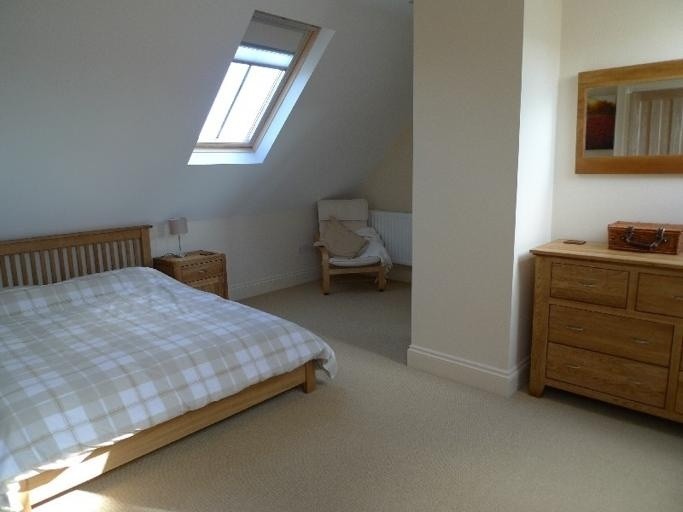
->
[316,198,385,296]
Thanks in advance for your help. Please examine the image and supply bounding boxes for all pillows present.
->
[312,215,369,260]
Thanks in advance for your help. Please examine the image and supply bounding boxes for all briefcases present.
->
[608,220,683,254]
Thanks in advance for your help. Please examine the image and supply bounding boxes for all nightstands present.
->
[152,249,229,300]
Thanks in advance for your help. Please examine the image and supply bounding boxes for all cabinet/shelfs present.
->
[527,238,683,423]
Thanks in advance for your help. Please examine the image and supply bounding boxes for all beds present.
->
[0,225,316,511]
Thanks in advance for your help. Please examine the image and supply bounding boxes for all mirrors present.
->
[573,60,683,175]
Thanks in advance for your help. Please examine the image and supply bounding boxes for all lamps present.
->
[168,217,194,259]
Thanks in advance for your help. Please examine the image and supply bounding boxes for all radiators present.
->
[366,210,412,267]
